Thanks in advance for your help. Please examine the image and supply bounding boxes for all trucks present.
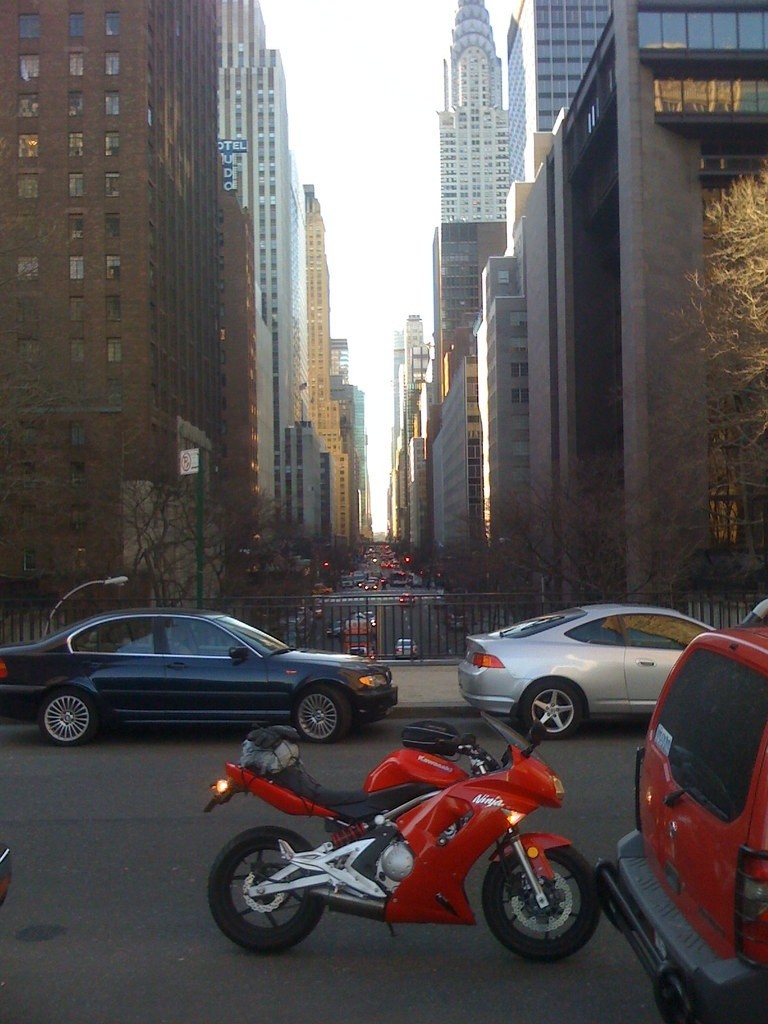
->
[342,620,375,656]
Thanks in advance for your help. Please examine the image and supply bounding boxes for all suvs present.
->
[594,596,767,1024]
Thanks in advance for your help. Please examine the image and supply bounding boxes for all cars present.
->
[310,542,417,638]
[396,639,418,657]
[0,608,396,749]
[458,601,717,738]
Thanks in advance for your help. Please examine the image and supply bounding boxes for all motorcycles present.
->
[204,711,604,967]
